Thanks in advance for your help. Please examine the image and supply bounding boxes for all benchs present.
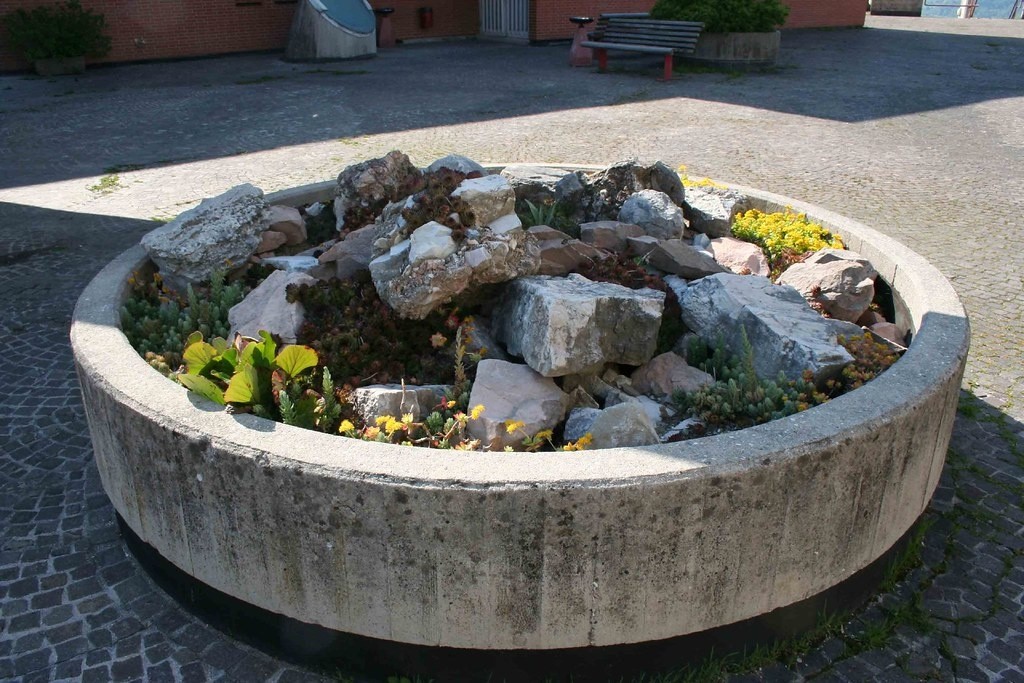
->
[586,13,650,60]
[579,19,705,80]
